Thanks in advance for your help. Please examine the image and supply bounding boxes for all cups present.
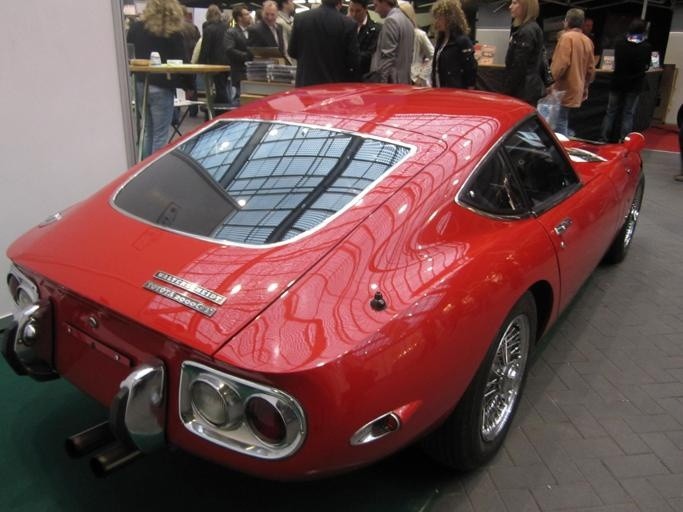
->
[150,52,161,67]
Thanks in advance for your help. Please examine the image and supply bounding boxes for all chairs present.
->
[203,73,239,121]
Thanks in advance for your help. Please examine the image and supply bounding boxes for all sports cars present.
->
[3,82,646,486]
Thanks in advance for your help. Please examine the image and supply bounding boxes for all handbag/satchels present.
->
[541,50,555,87]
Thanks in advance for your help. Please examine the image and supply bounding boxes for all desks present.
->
[128,62,231,162]
[169,98,204,142]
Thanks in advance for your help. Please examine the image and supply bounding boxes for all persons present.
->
[123,0,683,181]
